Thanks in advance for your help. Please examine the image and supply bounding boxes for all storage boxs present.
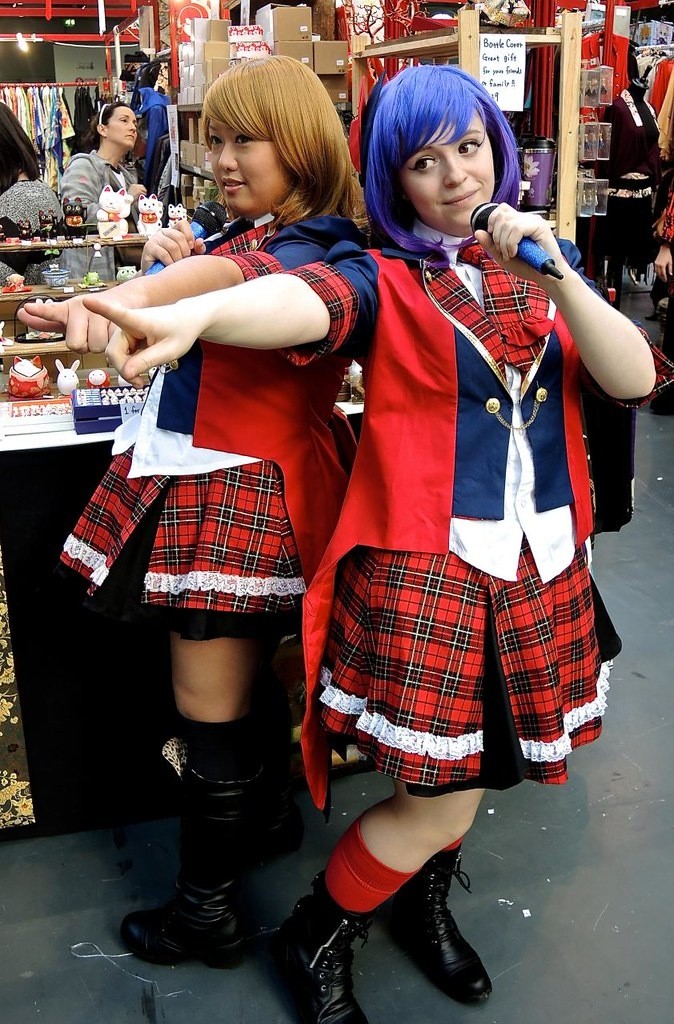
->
[177,7,350,174]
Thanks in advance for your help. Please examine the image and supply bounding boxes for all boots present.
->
[369,843,493,1005]
[119,737,264,970]
[244,675,304,868]
[266,868,370,1024]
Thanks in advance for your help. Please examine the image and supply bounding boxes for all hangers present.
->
[0,76,111,107]
[575,15,674,79]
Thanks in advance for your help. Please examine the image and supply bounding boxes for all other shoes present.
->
[645,315,658,321]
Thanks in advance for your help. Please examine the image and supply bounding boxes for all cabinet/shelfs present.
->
[350,9,582,252]
[0,233,149,359]
[177,100,354,228]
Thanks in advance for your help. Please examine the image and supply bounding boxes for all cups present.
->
[41,263,71,291]
[522,136,558,206]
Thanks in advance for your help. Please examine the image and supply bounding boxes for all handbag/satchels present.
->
[119,64,135,81]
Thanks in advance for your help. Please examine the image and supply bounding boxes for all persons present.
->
[60,101,149,286]
[600,73,656,298]
[644,165,674,418]
[0,101,68,289]
[18,52,368,966]
[79,62,657,1024]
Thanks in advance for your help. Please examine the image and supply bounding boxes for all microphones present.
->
[146,201,226,277]
[470,202,564,282]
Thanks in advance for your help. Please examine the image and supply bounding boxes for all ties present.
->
[460,243,553,346]
